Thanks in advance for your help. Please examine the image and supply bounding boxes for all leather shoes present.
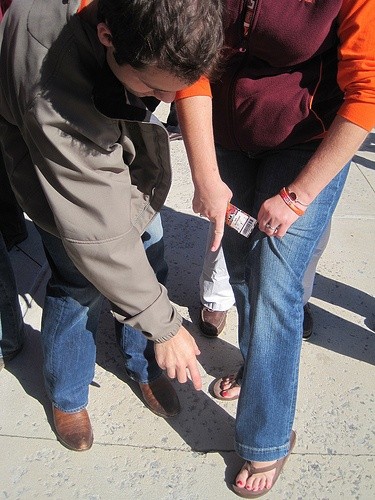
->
[52,402,94,451]
[139,374,181,418]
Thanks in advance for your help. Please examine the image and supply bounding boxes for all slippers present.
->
[231,430,296,498]
[212,374,241,401]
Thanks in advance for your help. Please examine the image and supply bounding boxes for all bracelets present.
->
[279,184,308,218]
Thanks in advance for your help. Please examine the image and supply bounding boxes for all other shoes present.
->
[2,231,28,252]
[168,132,182,141]
[198,302,227,338]
[301,303,313,341]
[0,342,24,372]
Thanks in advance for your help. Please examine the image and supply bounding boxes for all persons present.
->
[195,216,316,341]
[0,0,222,451]
[174,0,375,499]
